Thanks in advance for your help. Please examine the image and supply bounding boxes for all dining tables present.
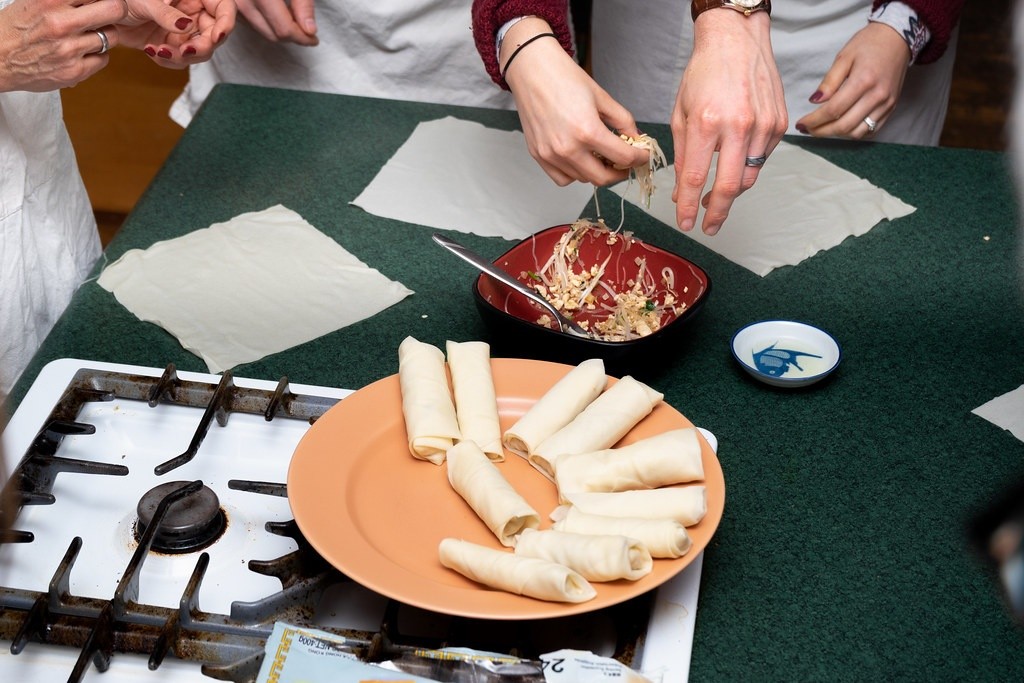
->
[1,80,1023,683]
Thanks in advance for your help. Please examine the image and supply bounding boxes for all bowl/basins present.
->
[471,223,712,390]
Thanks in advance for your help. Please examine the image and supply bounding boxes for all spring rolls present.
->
[397,332,707,602]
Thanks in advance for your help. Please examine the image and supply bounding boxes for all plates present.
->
[285,358,728,620]
[731,319,842,386]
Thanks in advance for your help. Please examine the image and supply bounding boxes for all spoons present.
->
[433,232,594,342]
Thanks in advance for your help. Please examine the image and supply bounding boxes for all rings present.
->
[744,154,766,168]
[864,116,877,133]
[93,29,109,55]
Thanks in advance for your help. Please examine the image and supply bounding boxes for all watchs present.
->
[690,0,772,24]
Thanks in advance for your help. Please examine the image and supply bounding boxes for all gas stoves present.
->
[0,356,720,683]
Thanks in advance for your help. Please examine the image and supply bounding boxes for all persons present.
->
[1,1,240,401]
[589,0,968,147]
[165,0,791,239]
